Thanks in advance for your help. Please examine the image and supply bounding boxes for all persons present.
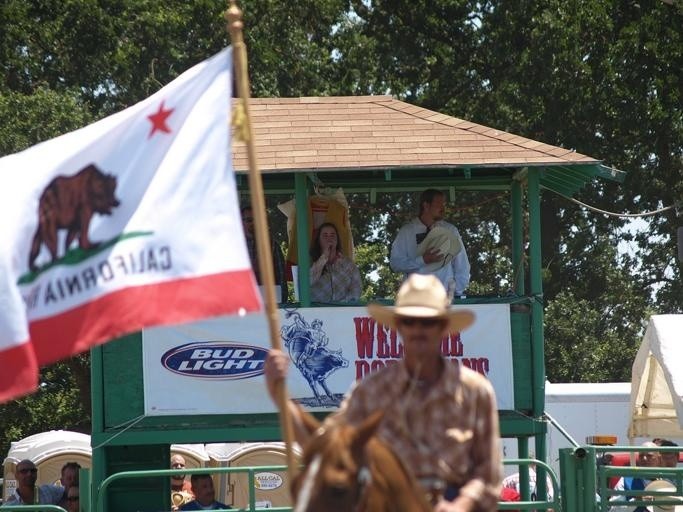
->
[264,273,504,512]
[60,462,81,505]
[66,481,79,512]
[502,451,552,512]
[0,459,65,505]
[389,188,471,299]
[241,206,289,303]
[659,441,683,485]
[180,474,232,510]
[608,441,676,512]
[170,454,196,511]
[310,222,362,302]
[296,319,329,367]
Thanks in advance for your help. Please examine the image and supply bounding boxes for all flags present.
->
[0,44,263,403]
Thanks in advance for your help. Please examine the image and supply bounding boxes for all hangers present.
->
[306,179,333,212]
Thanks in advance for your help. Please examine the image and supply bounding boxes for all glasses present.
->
[16,468,37,474]
[393,317,442,327]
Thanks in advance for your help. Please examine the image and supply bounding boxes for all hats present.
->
[416,226,463,272]
[642,479,683,511]
[368,273,475,337]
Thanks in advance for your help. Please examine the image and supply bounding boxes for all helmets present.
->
[311,319,322,328]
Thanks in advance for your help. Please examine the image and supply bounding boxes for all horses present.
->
[285,397,434,512]
